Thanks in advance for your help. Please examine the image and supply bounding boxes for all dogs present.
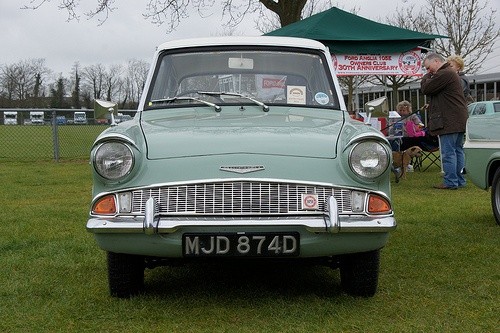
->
[390,145,423,183]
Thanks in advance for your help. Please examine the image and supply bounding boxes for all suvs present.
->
[56,116,67,126]
[67,114,132,125]
[23,119,30,126]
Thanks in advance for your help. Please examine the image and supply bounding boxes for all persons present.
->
[394,53,470,189]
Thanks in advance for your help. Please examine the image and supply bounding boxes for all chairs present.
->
[411,122,441,172]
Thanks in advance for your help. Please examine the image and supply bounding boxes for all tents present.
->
[259,6,451,113]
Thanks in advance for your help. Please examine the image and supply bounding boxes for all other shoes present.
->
[433,180,456,189]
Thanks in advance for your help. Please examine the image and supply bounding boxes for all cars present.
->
[462,99,500,226]
[85,36,397,298]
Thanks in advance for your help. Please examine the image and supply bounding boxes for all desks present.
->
[386,136,423,167]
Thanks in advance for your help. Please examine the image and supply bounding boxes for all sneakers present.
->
[395,165,414,173]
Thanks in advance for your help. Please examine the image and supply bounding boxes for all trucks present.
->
[30,111,45,126]
[3,111,18,126]
[74,111,87,126]
